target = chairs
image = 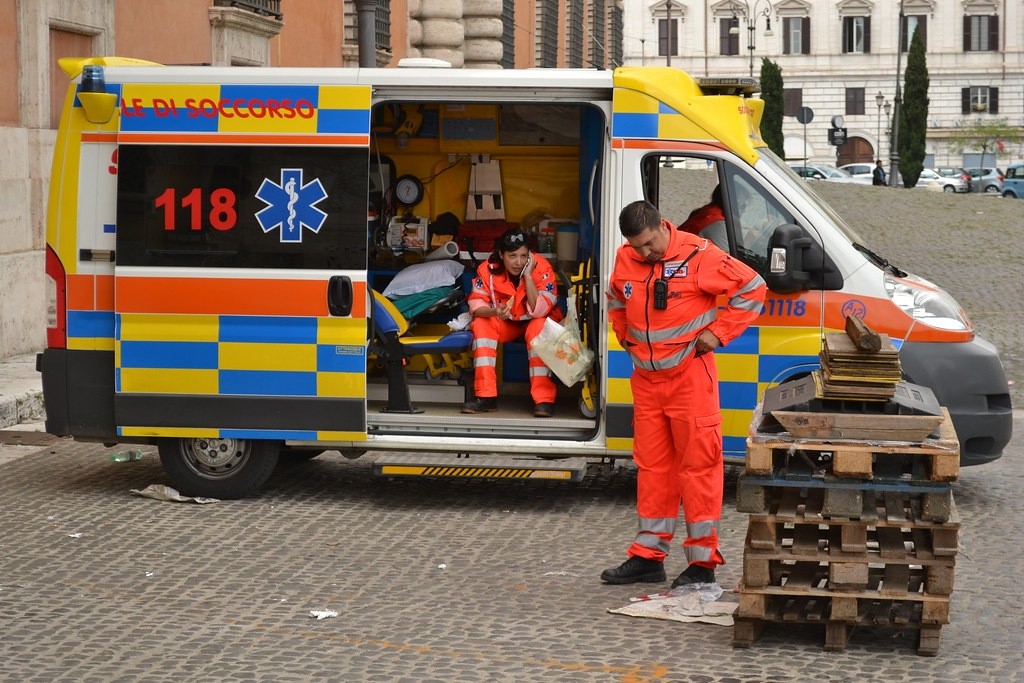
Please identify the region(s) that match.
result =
[369,285,472,383]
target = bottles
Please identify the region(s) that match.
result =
[544,236,553,253]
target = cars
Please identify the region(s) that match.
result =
[836,163,877,185]
[787,162,854,181]
[881,166,947,192]
[1001,162,1024,199]
[961,167,1005,194]
[933,167,970,193]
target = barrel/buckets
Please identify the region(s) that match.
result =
[555,226,579,261]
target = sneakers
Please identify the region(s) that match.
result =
[601,556,667,585]
[671,566,716,589]
[461,396,497,414]
[534,402,554,417]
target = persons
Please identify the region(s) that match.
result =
[873,160,886,186]
[463,229,563,417]
[677,180,750,253]
[604,201,767,590]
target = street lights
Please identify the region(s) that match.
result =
[640,33,649,65]
[875,91,884,161]
[883,100,892,161]
[729,1,775,78]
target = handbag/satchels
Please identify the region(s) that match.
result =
[531,305,596,388]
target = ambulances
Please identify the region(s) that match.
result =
[35,56,1014,501]
